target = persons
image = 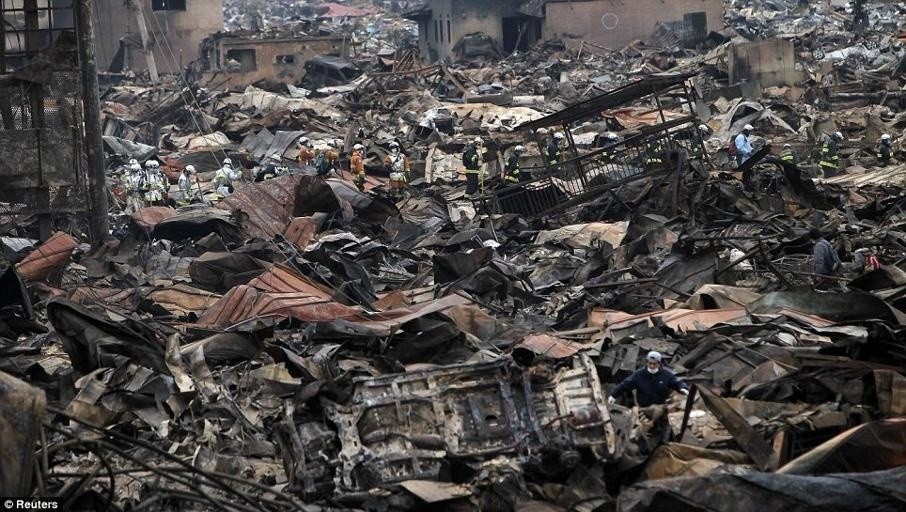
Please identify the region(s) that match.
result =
[120,158,204,214]
[294,133,345,177]
[821,129,845,180]
[212,157,244,196]
[810,133,828,162]
[733,124,756,167]
[608,350,690,444]
[779,142,796,163]
[383,141,411,194]
[259,153,287,183]
[646,138,663,164]
[351,142,369,192]
[874,135,896,165]
[464,135,485,199]
[501,144,526,188]
[690,122,714,161]
[804,225,843,284]
[545,129,566,178]
[595,143,628,166]
[796,155,825,179]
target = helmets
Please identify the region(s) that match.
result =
[129,132,618,174]
[698,124,792,149]
